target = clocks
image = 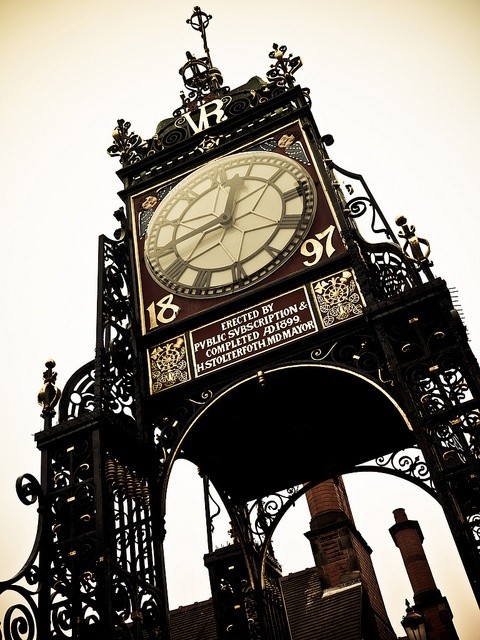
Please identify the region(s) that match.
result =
[129,117,350,336]
